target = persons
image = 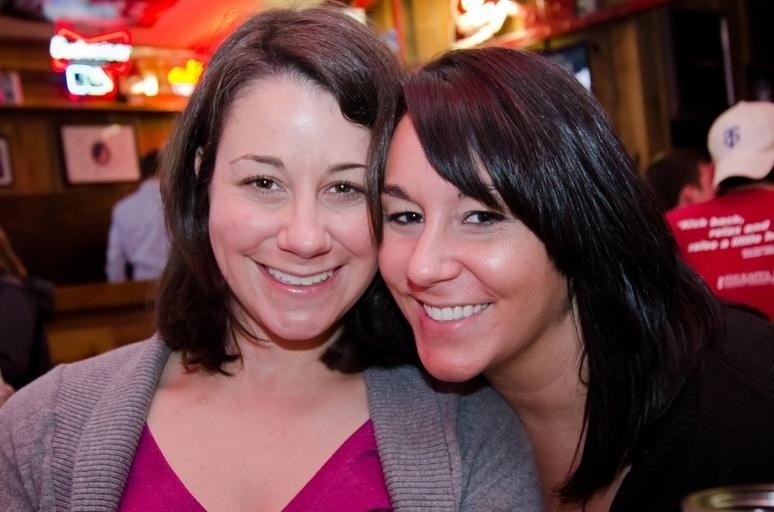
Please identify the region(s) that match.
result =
[105,148,176,282]
[364,44,774,512]
[646,148,716,219]
[665,96,774,326]
[2,6,548,512]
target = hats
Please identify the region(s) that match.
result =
[706,100,773,192]
